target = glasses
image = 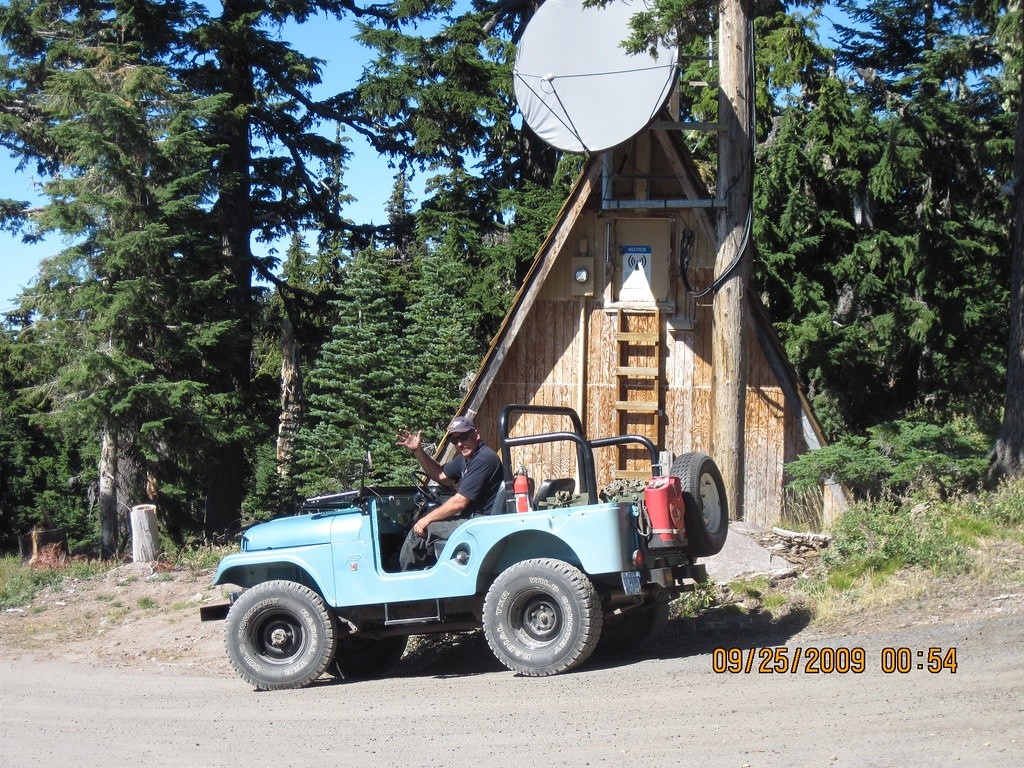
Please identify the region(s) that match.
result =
[450,430,474,445]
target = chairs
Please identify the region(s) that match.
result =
[531,478,576,512]
[432,477,535,561]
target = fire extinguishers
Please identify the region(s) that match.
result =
[513,464,533,513]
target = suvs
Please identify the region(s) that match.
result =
[197,404,731,692]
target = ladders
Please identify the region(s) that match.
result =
[613,307,661,482]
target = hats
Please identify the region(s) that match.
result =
[449,417,475,435]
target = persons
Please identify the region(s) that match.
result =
[393,417,504,572]
